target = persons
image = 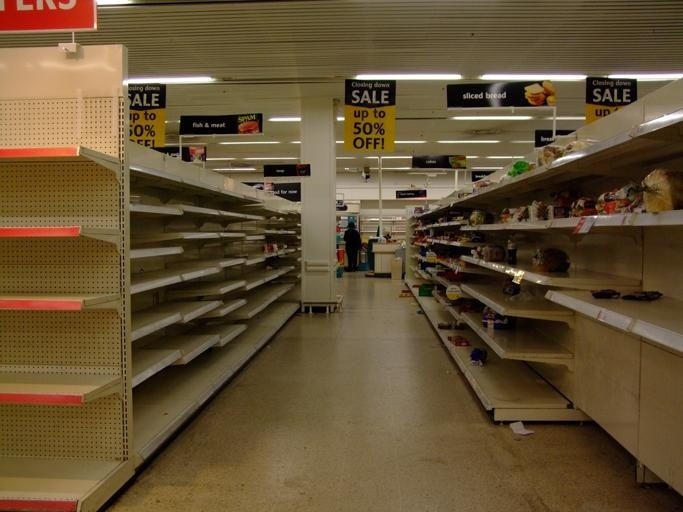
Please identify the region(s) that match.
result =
[344,222,362,272]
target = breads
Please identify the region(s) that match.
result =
[638,167,682,213]
[522,81,555,106]
[532,247,571,273]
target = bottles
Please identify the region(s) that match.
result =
[507,240,517,263]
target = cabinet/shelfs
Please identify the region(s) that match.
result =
[0,45,304,512]
[405,77,683,497]
[336,211,407,274]
